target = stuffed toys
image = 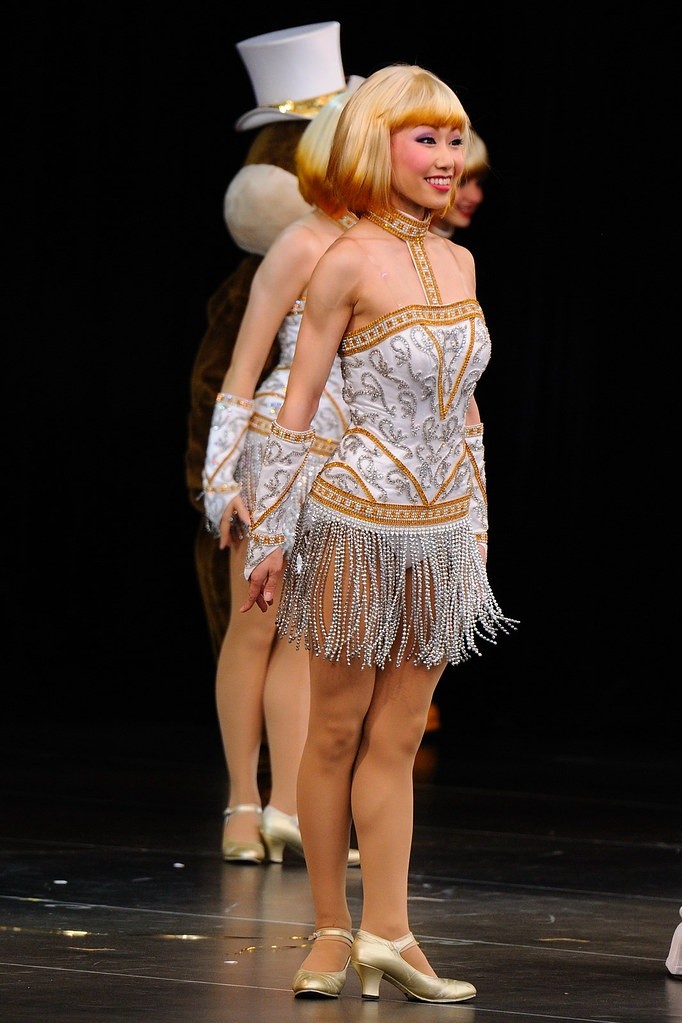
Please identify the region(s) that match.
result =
[184,20,346,657]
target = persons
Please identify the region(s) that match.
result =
[426,125,489,240]
[233,63,519,1004]
[197,87,366,870]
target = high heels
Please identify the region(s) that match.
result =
[291,925,355,998]
[352,929,477,1003]
[259,802,360,866]
[222,802,265,864]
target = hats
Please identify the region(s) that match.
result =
[236,20,351,132]
